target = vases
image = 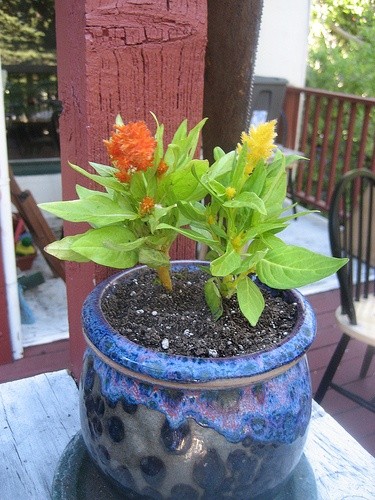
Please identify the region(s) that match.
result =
[79,260,318,500]
[17,247,37,271]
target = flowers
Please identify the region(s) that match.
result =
[37,109,348,328]
[16,237,35,256]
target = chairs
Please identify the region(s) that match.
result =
[4,81,62,157]
[272,110,298,221]
[315,168,375,412]
